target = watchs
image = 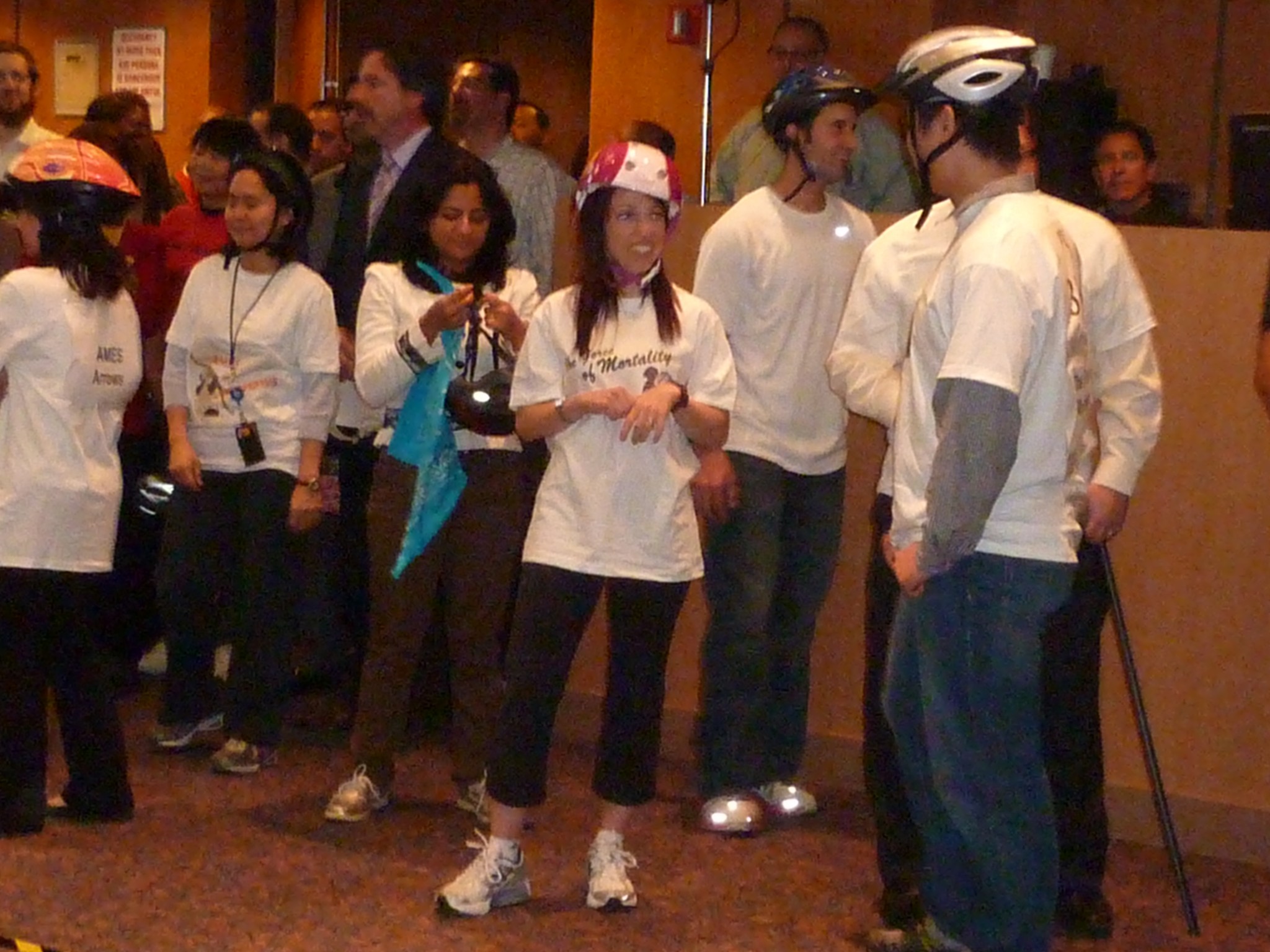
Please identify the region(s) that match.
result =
[300,478,322,491]
[556,399,576,424]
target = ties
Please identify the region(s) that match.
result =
[365,157,395,245]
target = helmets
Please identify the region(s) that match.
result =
[5,140,142,201]
[761,68,880,138]
[891,26,1037,109]
[574,139,683,219]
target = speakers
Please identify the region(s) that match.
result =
[1226,112,1270,232]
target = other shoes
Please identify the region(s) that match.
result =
[47,794,134,822]
[868,916,971,952]
[701,794,764,833]
[1057,888,1112,937]
[760,779,818,817]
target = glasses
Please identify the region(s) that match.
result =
[766,45,823,64]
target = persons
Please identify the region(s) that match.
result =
[1092,122,1202,226]
[705,17,915,210]
[324,149,543,828]
[828,103,1161,937]
[157,149,340,774]
[0,140,145,836]
[882,27,1082,952]
[0,35,580,726]
[691,65,880,826]
[432,139,738,913]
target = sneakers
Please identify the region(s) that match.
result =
[159,707,222,749]
[211,737,277,774]
[435,828,533,920]
[457,773,495,824]
[325,766,388,824]
[585,827,639,910]
